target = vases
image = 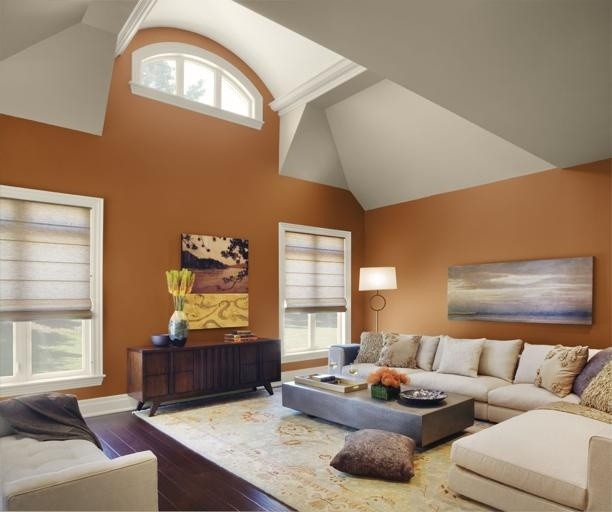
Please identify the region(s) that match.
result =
[168,311,189,347]
[371,384,401,400]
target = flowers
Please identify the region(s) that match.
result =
[364,368,411,389]
[166,268,195,311]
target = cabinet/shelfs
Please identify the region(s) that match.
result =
[128,338,281,416]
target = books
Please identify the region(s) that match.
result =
[224,330,258,344]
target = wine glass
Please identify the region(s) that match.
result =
[348,361,358,379]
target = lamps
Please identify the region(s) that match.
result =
[358,267,397,332]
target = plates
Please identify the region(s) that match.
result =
[399,389,449,404]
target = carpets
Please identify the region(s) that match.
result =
[131,388,506,512]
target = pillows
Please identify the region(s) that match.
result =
[329,427,417,483]
[581,362,612,415]
[437,336,485,378]
[514,342,602,384]
[535,344,589,397]
[571,347,612,398]
[478,339,522,382]
[375,332,422,368]
[354,332,383,363]
[414,336,440,372]
[432,335,445,371]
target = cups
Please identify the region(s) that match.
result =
[329,363,338,377]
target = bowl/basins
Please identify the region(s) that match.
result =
[151,334,170,346]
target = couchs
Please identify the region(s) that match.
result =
[446,406,612,512]
[329,332,612,421]
[0,394,159,512]
